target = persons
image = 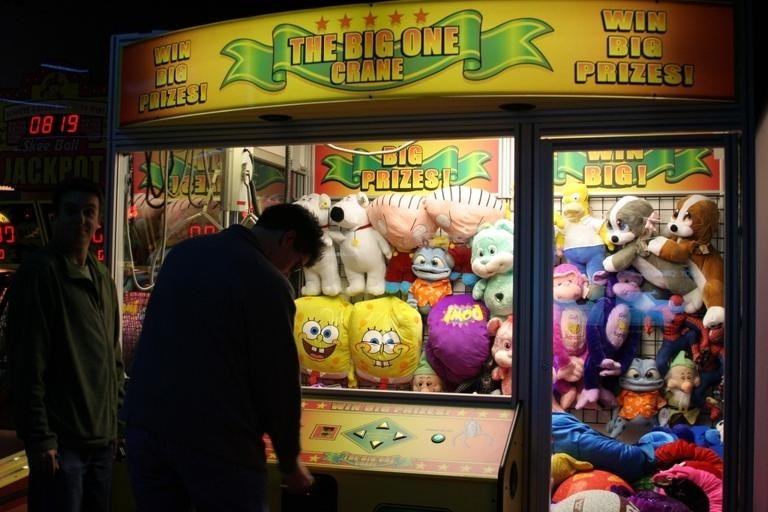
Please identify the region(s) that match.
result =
[2,177,130,510]
[120,200,327,510]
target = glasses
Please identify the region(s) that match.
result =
[290,243,303,273]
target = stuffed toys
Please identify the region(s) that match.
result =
[290,180,513,396]
[290,180,512,396]
[551,183,722,512]
[551,174,723,512]
